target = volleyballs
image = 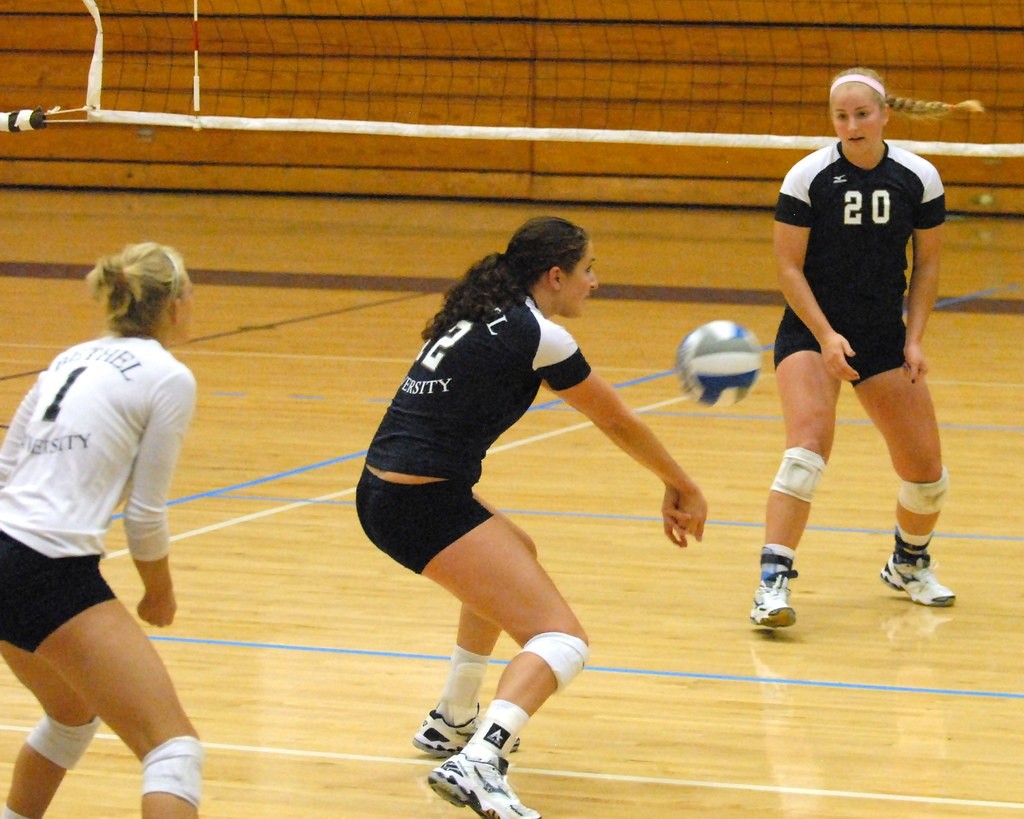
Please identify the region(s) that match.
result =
[674,319,765,409]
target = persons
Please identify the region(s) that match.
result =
[357,215,706,817]
[1,241,198,819]
[746,66,982,631]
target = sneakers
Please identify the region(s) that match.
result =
[750,569,799,627]
[413,708,521,756]
[880,551,956,607]
[427,752,540,819]
